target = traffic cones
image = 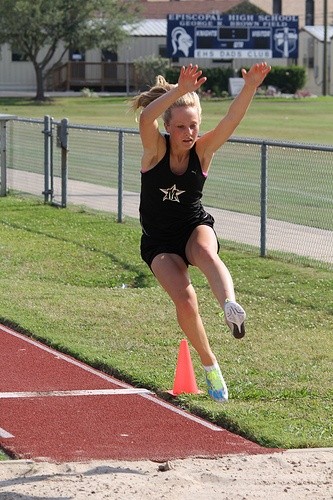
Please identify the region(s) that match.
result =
[166,339,209,401]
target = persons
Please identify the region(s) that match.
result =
[131,61,273,402]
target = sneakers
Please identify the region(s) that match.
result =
[222,301,246,338]
[200,355,228,403]
